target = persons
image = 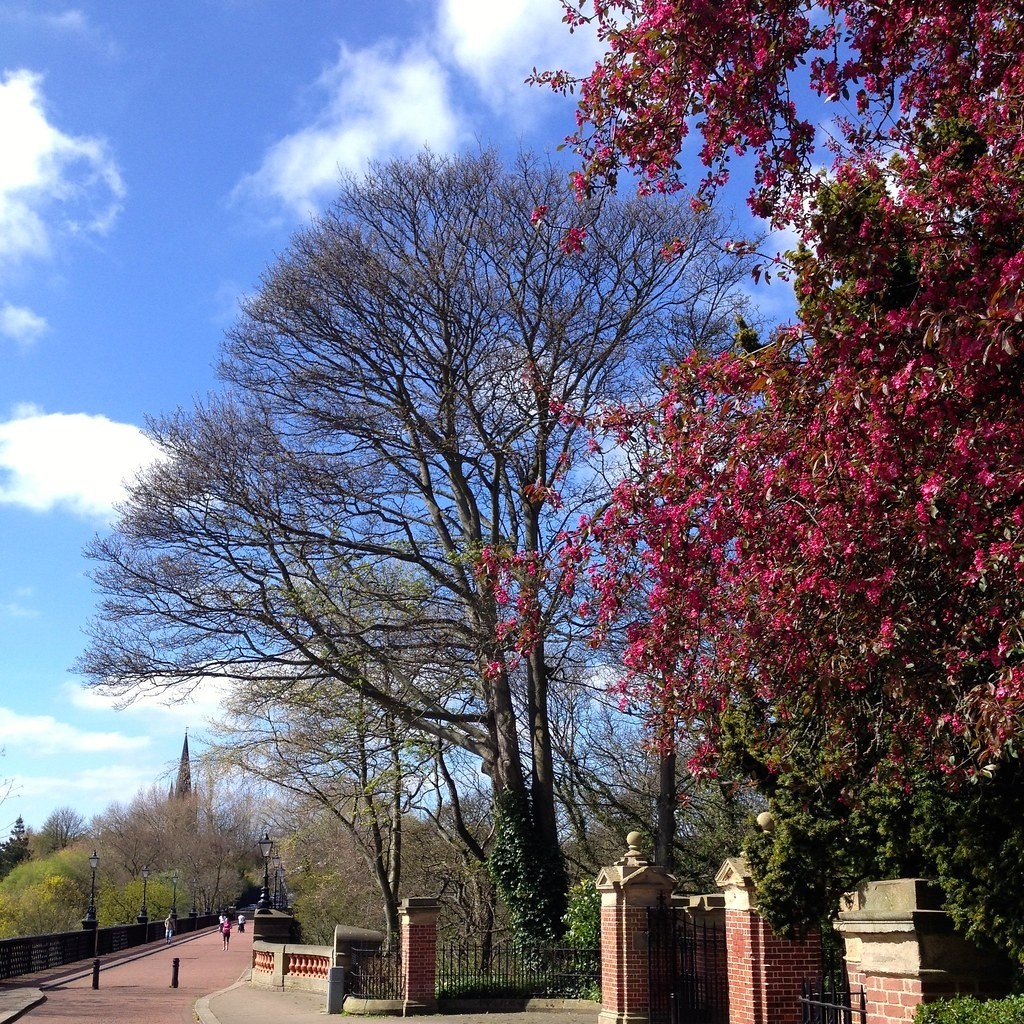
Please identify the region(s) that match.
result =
[238,913,245,933]
[219,912,226,934]
[165,913,175,944]
[222,918,232,951]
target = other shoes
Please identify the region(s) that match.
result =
[166,941,171,944]
[222,948,228,951]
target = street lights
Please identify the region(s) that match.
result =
[169,872,179,916]
[85,849,101,921]
[257,832,274,908]
[139,863,149,916]
[271,849,288,910]
[190,876,199,912]
